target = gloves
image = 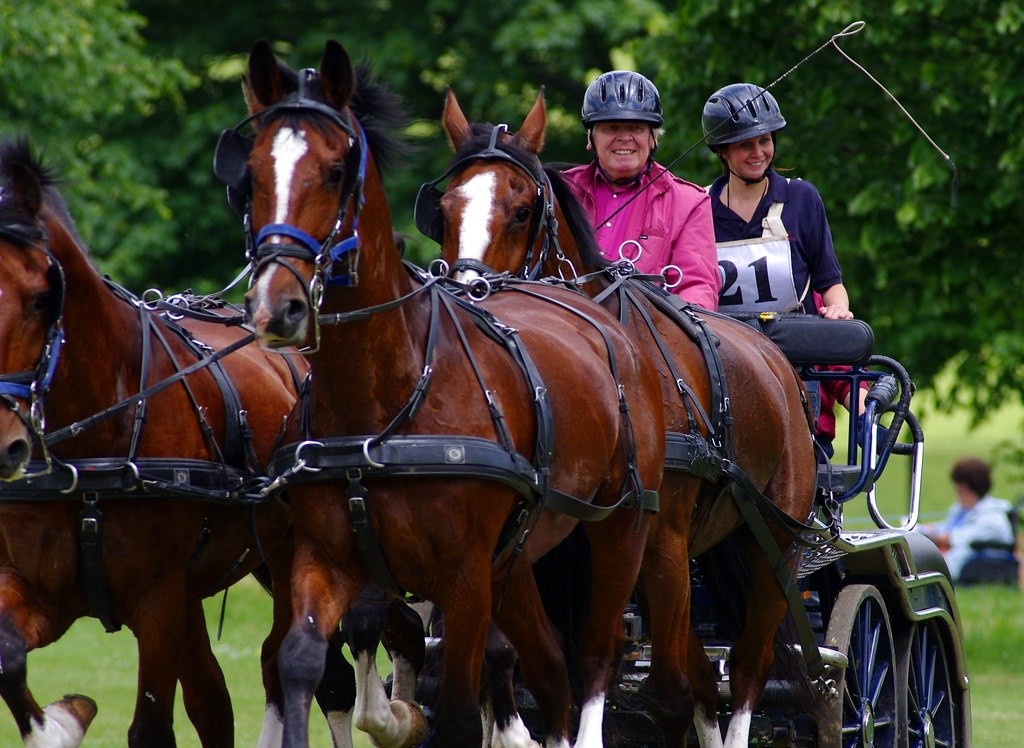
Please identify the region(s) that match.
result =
[857,413,888,455]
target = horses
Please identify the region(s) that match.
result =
[438,80,815,748]
[215,37,661,745]
[1,128,429,748]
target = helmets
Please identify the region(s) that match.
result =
[701,84,786,153]
[581,71,664,130]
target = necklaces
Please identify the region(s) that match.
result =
[723,176,768,208]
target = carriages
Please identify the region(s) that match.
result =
[0,39,974,747]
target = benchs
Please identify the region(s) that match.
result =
[762,318,875,493]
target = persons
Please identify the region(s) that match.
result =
[915,459,1024,586]
[697,81,855,423]
[554,70,720,315]
[814,290,868,464]
